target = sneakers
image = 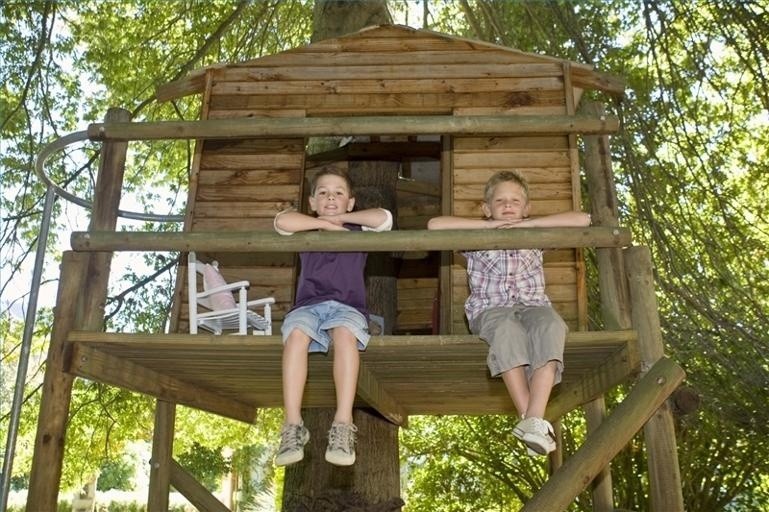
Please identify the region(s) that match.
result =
[325,420,358,466]
[275,420,310,467]
[512,417,556,456]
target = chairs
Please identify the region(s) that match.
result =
[187,252,276,336]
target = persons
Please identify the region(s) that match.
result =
[426,169,592,457]
[272,163,394,467]
